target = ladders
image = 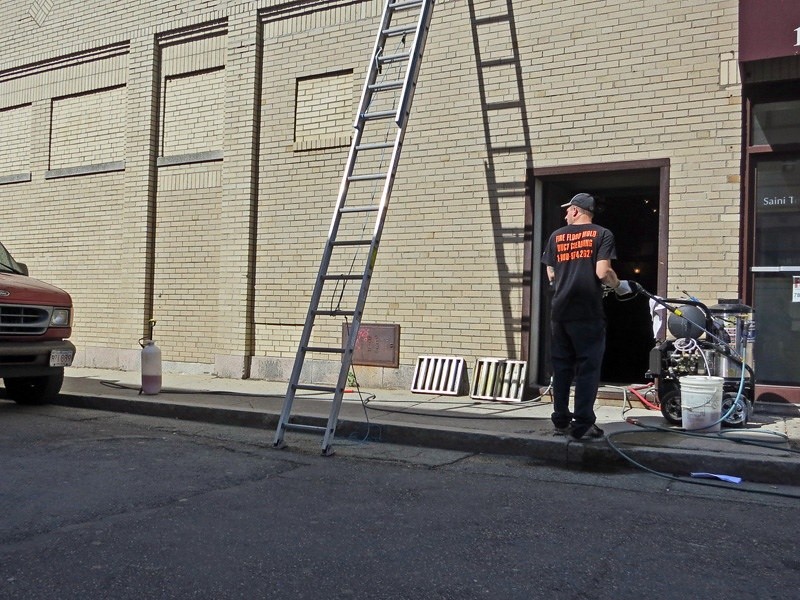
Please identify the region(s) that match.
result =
[274,0,434,456]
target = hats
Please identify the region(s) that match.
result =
[561,193,595,213]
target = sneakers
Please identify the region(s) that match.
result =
[581,423,604,439]
[554,423,572,435]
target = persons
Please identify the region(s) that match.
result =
[541,193,633,437]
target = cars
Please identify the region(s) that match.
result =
[0,241,75,405]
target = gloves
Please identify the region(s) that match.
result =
[614,280,633,296]
